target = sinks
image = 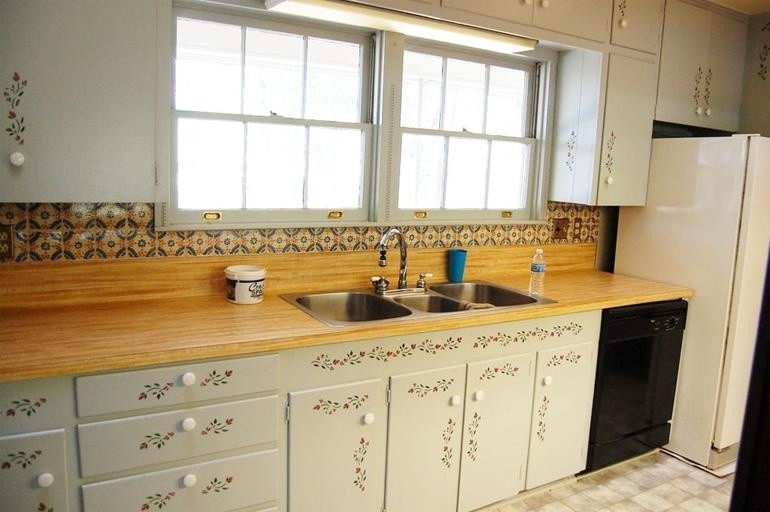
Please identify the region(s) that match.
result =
[391,290,476,314]
[288,288,417,326]
[427,280,539,307]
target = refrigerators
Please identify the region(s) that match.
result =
[614,133,769,474]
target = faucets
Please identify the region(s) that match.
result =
[377,227,409,288]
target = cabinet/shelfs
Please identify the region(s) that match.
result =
[0,0,172,203]
[353,0,664,60]
[524,311,603,491]
[749,11,769,136]
[547,48,660,209]
[655,3,747,132]
[75,350,289,512]
[0,376,78,512]
[288,337,389,511]
[388,318,538,512]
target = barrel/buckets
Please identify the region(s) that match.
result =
[223,264,267,304]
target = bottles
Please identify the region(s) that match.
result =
[529,248,545,296]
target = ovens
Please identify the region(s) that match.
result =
[582,295,691,481]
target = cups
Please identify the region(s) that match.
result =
[447,249,467,283]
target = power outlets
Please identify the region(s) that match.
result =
[574,217,581,239]
[553,218,568,240]
